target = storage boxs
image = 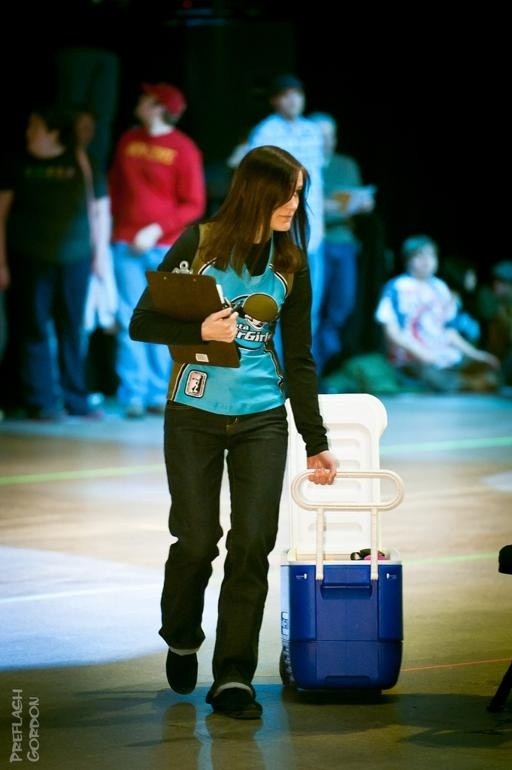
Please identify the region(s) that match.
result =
[278,391,406,703]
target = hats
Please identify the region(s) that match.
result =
[141,83,187,118]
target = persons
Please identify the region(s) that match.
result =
[125,144,340,721]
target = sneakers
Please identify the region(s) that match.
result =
[211,685,263,719]
[164,646,198,695]
